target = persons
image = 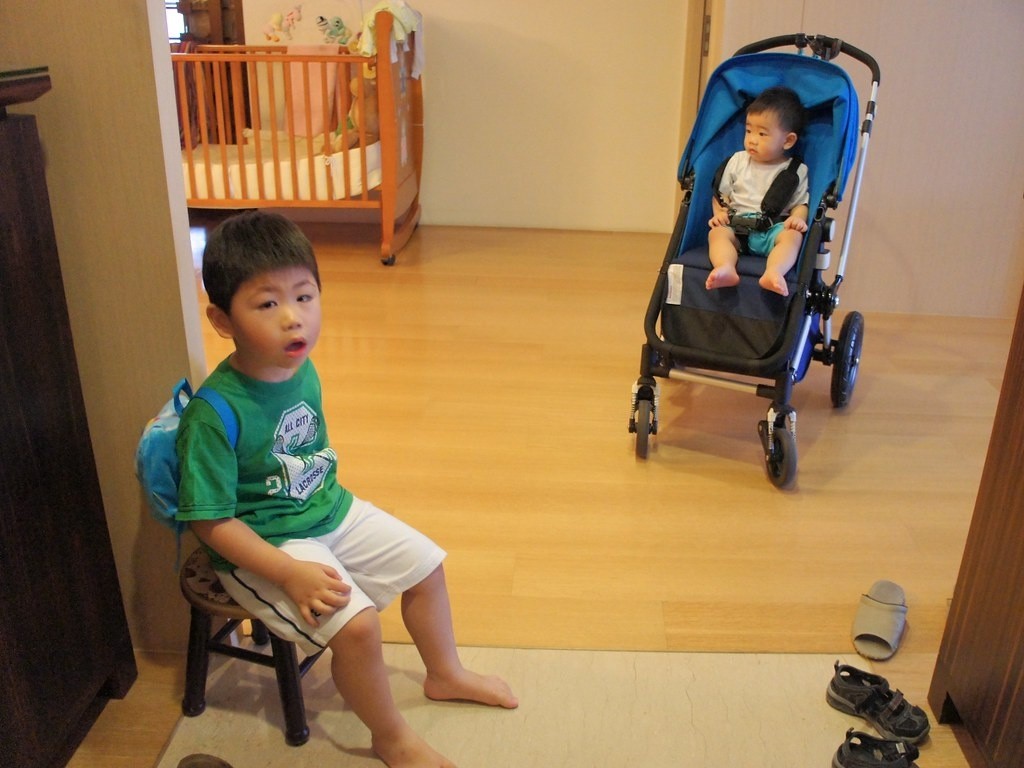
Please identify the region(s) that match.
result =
[704,84,812,294]
[170,208,521,768]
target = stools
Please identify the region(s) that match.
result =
[179,545,310,747]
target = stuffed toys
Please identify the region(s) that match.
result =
[323,61,381,155]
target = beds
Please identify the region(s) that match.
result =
[165,0,425,265]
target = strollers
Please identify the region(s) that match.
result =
[626,31,883,490]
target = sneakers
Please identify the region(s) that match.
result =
[827,662,931,768]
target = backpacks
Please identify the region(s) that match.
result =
[137,377,240,531]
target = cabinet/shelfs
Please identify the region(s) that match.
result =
[0,65,142,767]
[927,287,1024,768]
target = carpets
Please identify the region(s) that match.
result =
[150,640,885,767]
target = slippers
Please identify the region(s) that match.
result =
[851,581,908,661]
